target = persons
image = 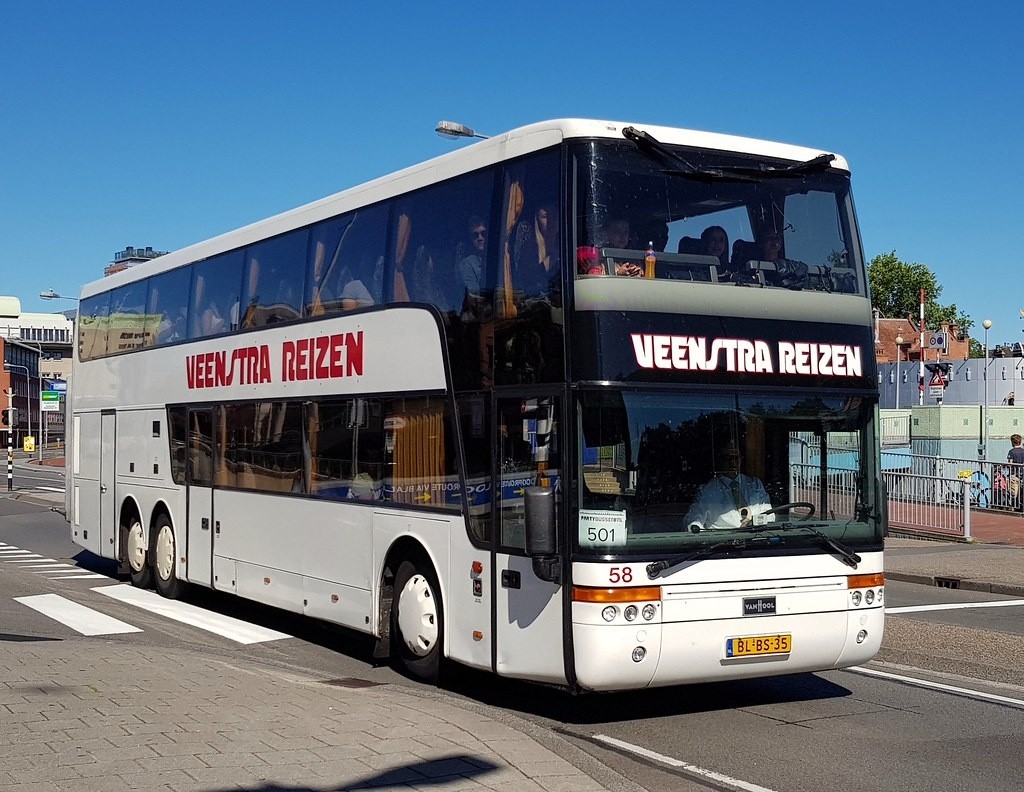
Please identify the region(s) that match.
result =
[1007,434,1024,512]
[682,435,776,531]
[351,472,384,500]
[156,201,784,344]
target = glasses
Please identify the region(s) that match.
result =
[471,231,487,239]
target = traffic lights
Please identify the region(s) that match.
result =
[926,363,952,373]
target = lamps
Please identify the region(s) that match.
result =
[903,359,918,382]
[985,348,1004,371]
[957,344,982,374]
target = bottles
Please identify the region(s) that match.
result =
[645,241,656,279]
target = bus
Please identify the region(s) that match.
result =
[65,116,885,693]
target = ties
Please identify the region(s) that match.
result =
[731,481,752,520]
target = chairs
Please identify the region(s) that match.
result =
[168,235,754,337]
[192,432,382,499]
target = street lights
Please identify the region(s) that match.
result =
[4,363,31,436]
[895,337,903,409]
[982,319,992,461]
[8,336,42,467]
